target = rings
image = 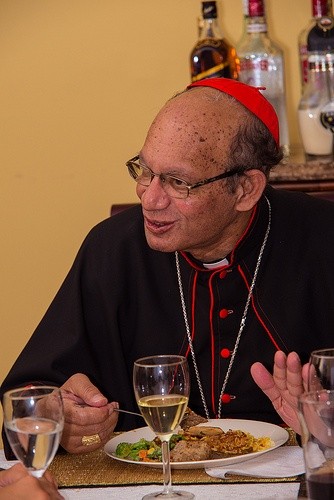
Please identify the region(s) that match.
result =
[82,434,102,446]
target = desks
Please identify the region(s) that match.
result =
[0,423,334,500]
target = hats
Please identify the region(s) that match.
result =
[187,77,279,147]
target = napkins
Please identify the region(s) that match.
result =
[206,441,326,480]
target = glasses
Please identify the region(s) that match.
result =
[126,155,238,199]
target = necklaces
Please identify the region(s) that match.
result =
[175,196,271,419]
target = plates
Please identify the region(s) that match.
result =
[104,419,290,469]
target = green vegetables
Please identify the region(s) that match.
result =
[115,434,183,460]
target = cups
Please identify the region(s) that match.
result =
[307,348,334,430]
[3,386,64,479]
[296,390,334,500]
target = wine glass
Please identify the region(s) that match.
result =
[133,355,195,500]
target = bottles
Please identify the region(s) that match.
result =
[189,0,240,83]
[298,0,334,156]
[237,0,291,165]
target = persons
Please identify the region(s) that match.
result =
[0,79,334,463]
